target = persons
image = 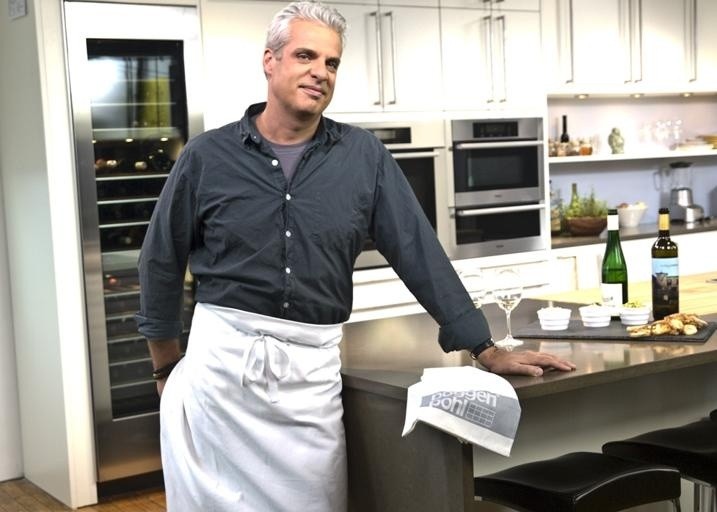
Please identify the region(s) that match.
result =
[133,1,577,512]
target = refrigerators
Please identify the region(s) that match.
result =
[60,4,211,489]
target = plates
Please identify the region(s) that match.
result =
[694,134,716,150]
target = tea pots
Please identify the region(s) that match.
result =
[667,119,685,152]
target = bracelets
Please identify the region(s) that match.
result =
[469,337,493,361]
[153,354,184,380]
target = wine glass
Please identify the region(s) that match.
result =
[455,269,488,312]
[488,269,526,348]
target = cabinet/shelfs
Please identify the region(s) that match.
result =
[601,1,661,95]
[313,1,445,117]
[439,0,547,117]
[346,265,434,325]
[661,1,716,95]
[543,0,600,95]
[457,258,550,298]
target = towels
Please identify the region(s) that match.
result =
[399,365,522,458]
[574,254,602,290]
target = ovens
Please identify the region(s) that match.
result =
[447,118,547,255]
[351,122,451,270]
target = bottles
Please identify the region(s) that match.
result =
[600,207,626,314]
[652,207,678,318]
[569,137,580,156]
[579,136,592,155]
[548,137,555,157]
[94,141,182,174]
[553,138,559,157]
[560,116,570,156]
[549,179,564,234]
[100,270,121,289]
[567,183,579,209]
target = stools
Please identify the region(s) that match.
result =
[604,413,716,511]
[473,445,681,510]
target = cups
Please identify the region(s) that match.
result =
[578,305,610,328]
[616,303,652,326]
[536,307,572,331]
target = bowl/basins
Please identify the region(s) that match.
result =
[616,204,647,228]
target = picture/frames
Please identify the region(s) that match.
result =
[6,0,27,21]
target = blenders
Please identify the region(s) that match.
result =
[666,162,702,222]
